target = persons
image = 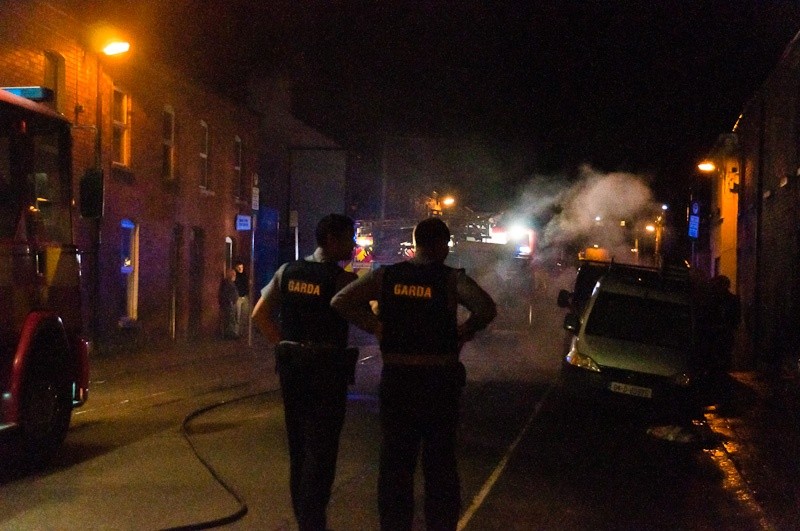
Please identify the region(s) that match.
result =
[251,214,375,530]
[710,275,740,417]
[330,218,497,531]
[218,260,250,340]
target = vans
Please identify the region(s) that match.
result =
[558,255,719,424]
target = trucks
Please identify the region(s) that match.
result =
[0,86,103,479]
